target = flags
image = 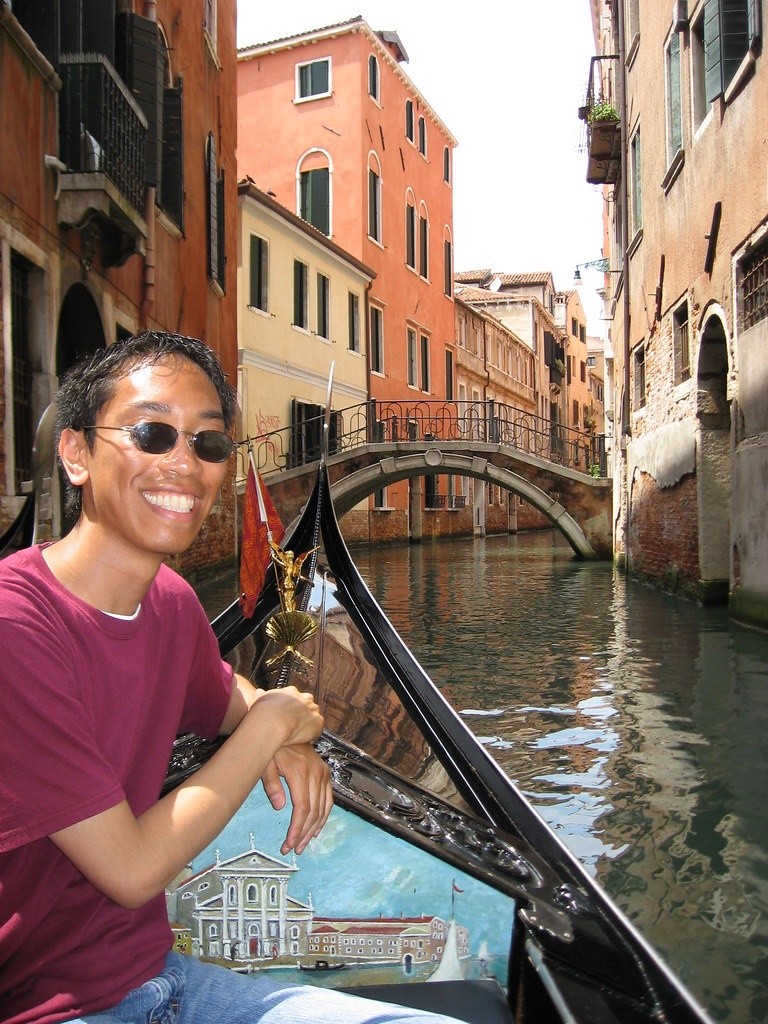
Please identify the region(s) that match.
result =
[238,460,286,619]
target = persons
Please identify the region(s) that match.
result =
[270,551,295,612]
[0,330,470,1024]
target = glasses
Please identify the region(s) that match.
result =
[79,421,240,464]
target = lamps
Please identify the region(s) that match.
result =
[572,258,609,286]
